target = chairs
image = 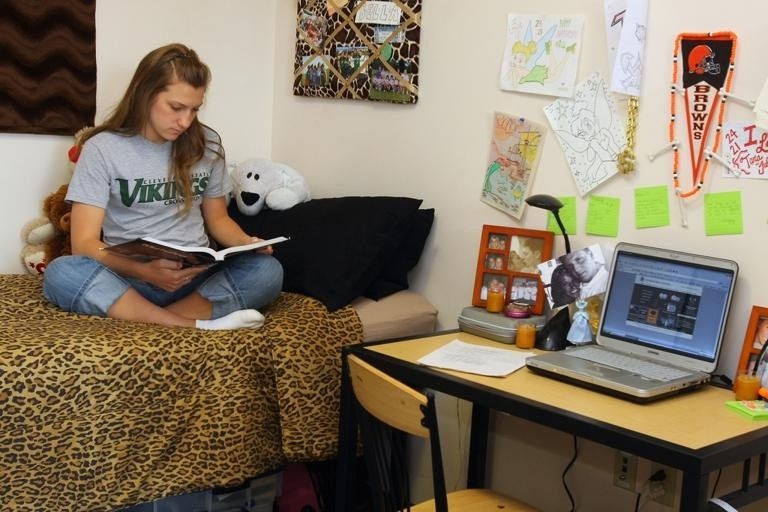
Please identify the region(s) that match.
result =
[341,348,544,512]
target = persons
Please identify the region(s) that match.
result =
[560,248,610,299]
[41,42,283,331]
[480,231,543,302]
[746,354,759,375]
[752,315,768,350]
[551,263,581,309]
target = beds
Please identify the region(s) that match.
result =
[2,272,438,512]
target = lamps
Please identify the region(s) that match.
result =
[525,195,573,353]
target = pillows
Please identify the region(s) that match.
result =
[227,188,435,315]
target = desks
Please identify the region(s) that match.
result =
[358,329,768,512]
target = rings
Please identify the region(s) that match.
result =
[185,275,190,281]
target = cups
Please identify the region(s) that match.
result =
[733,372,761,403]
[485,280,507,314]
[515,322,538,350]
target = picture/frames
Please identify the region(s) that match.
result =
[471,224,554,317]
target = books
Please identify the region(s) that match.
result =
[98,236,291,265]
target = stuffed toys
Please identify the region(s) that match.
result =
[18,126,94,282]
[225,157,313,219]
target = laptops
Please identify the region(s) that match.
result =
[525,242,739,404]
